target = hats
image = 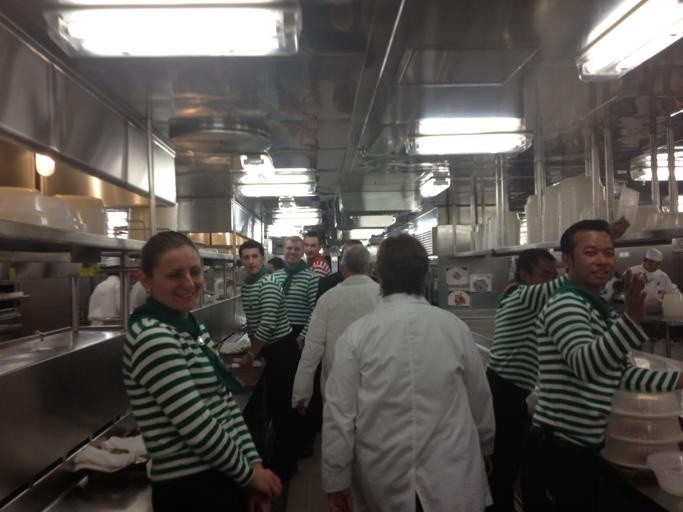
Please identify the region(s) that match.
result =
[646,248,663,261]
[104,257,120,266]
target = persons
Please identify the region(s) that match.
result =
[319,234,496,512]
[522,221,649,512]
[622,247,681,305]
[129,280,148,314]
[240,238,301,441]
[486,216,631,512]
[122,231,282,512]
[262,230,378,358]
[87,257,121,325]
[214,279,236,300]
[290,243,382,416]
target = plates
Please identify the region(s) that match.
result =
[75,453,152,483]
